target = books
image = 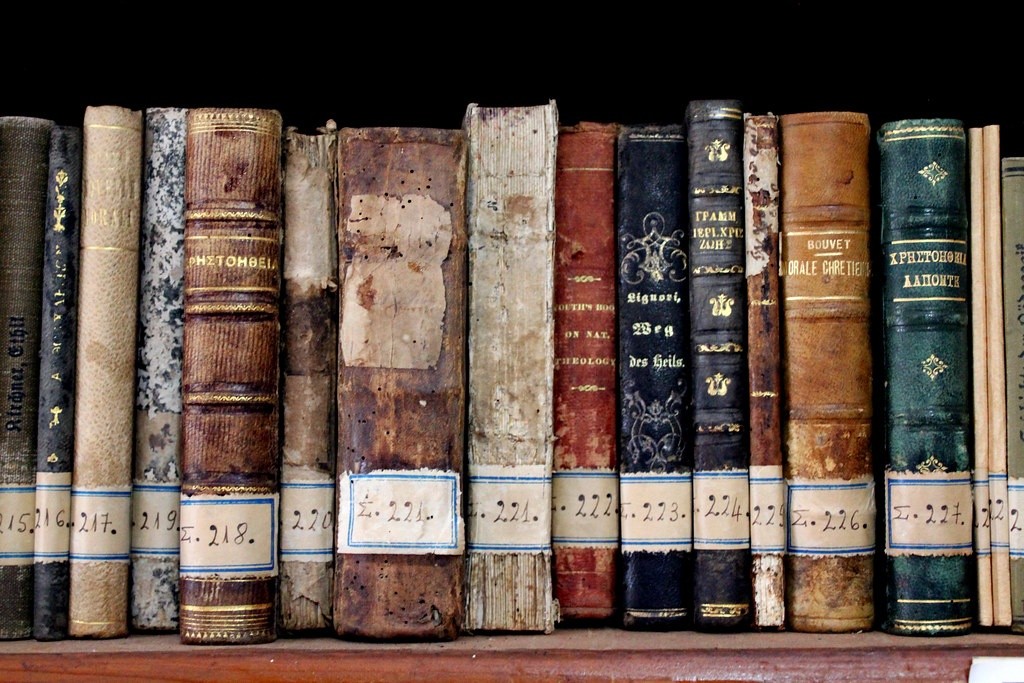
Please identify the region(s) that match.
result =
[1,103,1024,646]
